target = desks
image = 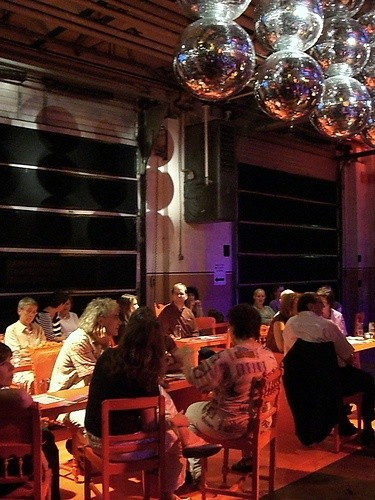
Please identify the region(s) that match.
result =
[175,333,227,374]
[346,337,375,352]
[31,374,195,416]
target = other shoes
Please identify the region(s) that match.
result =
[183,438,222,457]
[341,424,359,436]
[360,429,375,442]
[232,458,252,473]
[173,477,208,498]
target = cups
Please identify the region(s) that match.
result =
[369,322,375,339]
[175,325,181,340]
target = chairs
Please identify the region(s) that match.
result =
[0,303,363,500]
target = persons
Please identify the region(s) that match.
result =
[34,291,69,343]
[57,293,79,336]
[266,289,297,354]
[316,288,347,336]
[186,286,203,318]
[85,305,224,500]
[113,293,139,343]
[47,297,122,391]
[158,282,199,338]
[253,288,276,322]
[270,285,288,314]
[186,305,278,497]
[0,343,60,500]
[3,297,68,429]
[284,293,375,447]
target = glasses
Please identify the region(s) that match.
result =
[22,309,38,316]
[316,301,322,305]
